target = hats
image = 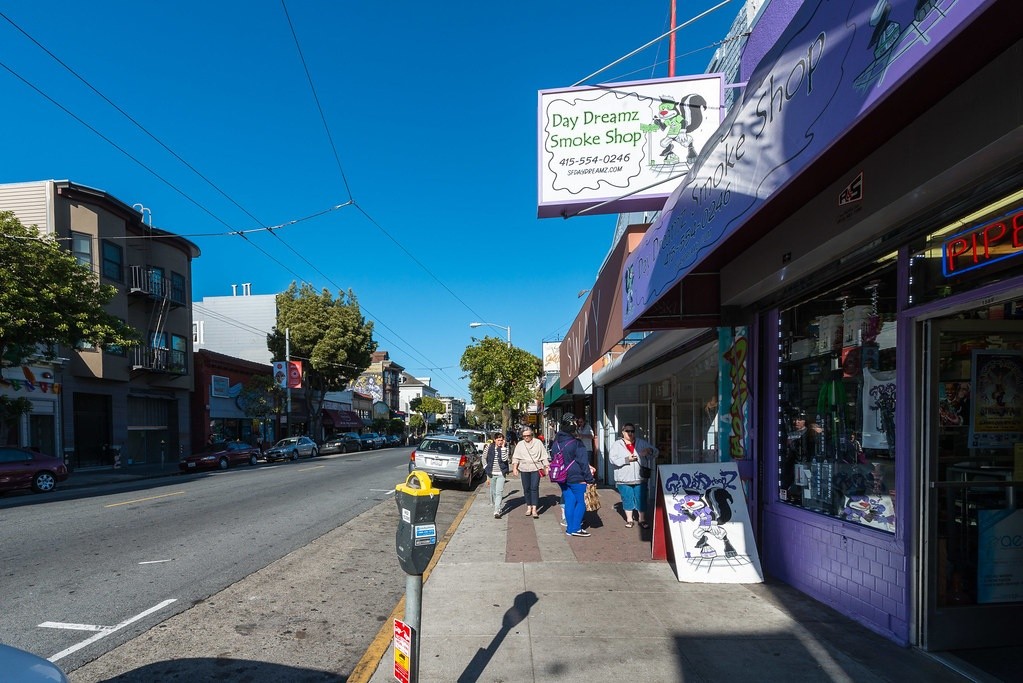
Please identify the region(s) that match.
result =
[561,418,576,432]
[563,413,578,421]
[523,427,533,436]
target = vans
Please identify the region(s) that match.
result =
[453,428,487,453]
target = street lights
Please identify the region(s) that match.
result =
[470,322,511,348]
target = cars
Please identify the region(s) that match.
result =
[382,435,401,448]
[178,440,263,470]
[325,432,362,453]
[408,435,485,489]
[263,436,319,461]
[0,445,69,493]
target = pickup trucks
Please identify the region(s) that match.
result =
[360,433,386,450]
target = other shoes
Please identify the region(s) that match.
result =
[494,511,500,518]
[532,511,538,518]
[525,510,532,515]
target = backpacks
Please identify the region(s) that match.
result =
[548,438,577,483]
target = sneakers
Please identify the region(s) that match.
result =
[566,529,591,536]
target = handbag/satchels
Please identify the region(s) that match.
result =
[639,466,652,478]
[538,469,546,477]
[584,483,602,512]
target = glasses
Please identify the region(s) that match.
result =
[625,430,635,433]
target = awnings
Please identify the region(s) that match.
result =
[354,392,374,401]
[321,409,363,427]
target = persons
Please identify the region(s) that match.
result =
[940,381,971,417]
[257,435,263,451]
[786,410,820,500]
[378,430,382,437]
[512,427,549,517]
[208,434,216,448]
[292,428,314,441]
[551,413,595,536]
[482,432,509,518]
[850,432,863,456]
[703,396,718,450]
[224,436,233,442]
[609,423,659,530]
[359,427,362,436]
[808,422,826,461]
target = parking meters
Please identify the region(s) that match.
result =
[395,470,441,683]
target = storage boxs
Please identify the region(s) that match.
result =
[790,337,817,361]
[818,313,843,352]
[842,304,872,377]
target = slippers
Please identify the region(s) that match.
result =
[625,521,634,528]
[637,520,649,529]
